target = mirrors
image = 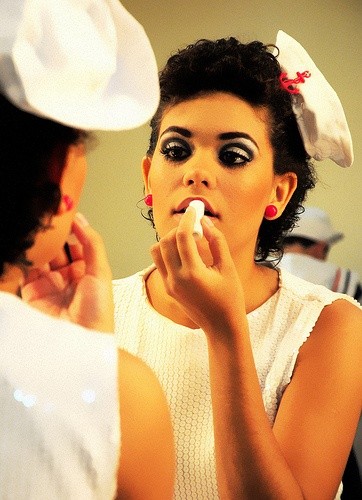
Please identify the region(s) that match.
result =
[75,0,362,500]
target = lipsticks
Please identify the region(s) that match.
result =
[187,200,206,239]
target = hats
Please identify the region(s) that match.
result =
[273,28,354,170]
[281,207,345,244]
[0,0,159,133]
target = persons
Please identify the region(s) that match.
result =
[110,30,362,500]
[1,0,177,500]
